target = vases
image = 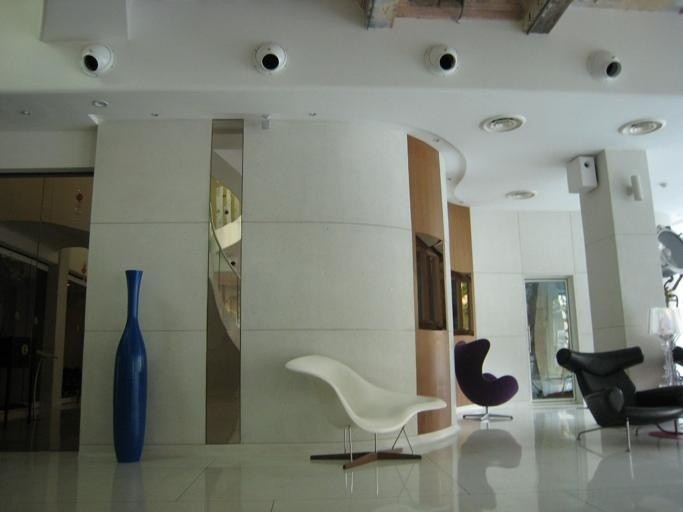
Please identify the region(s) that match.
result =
[110,267,149,464]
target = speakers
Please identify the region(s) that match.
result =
[567,155,598,193]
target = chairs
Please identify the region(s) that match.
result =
[555,338,682,460]
[453,333,518,426]
[283,344,450,473]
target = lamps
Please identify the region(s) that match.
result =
[624,173,642,203]
[646,303,683,440]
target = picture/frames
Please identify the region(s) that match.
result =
[450,269,475,336]
[413,232,447,332]
